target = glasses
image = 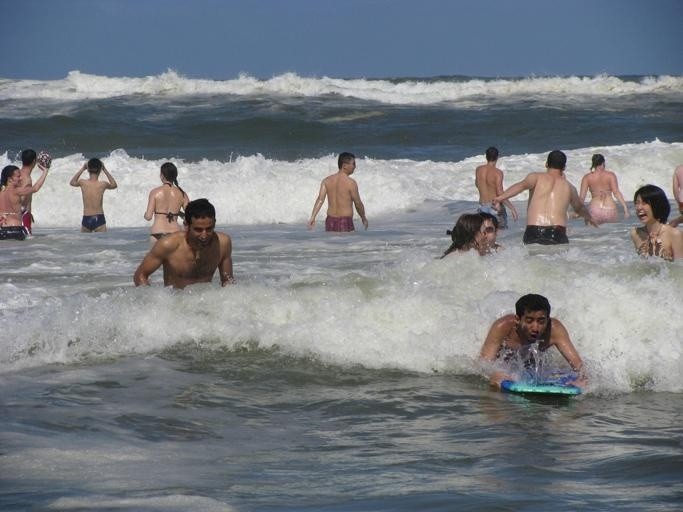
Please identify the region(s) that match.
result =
[486,226,495,234]
[344,162,356,165]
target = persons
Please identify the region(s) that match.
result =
[669,216,682,228]
[482,293,586,395]
[1,161,52,239]
[474,147,521,221]
[441,214,489,259]
[17,148,35,235]
[70,157,118,232]
[472,213,501,252]
[145,162,189,241]
[133,199,234,291]
[668,165,683,227]
[493,149,599,245]
[632,185,683,261]
[307,153,370,234]
[580,154,629,224]
